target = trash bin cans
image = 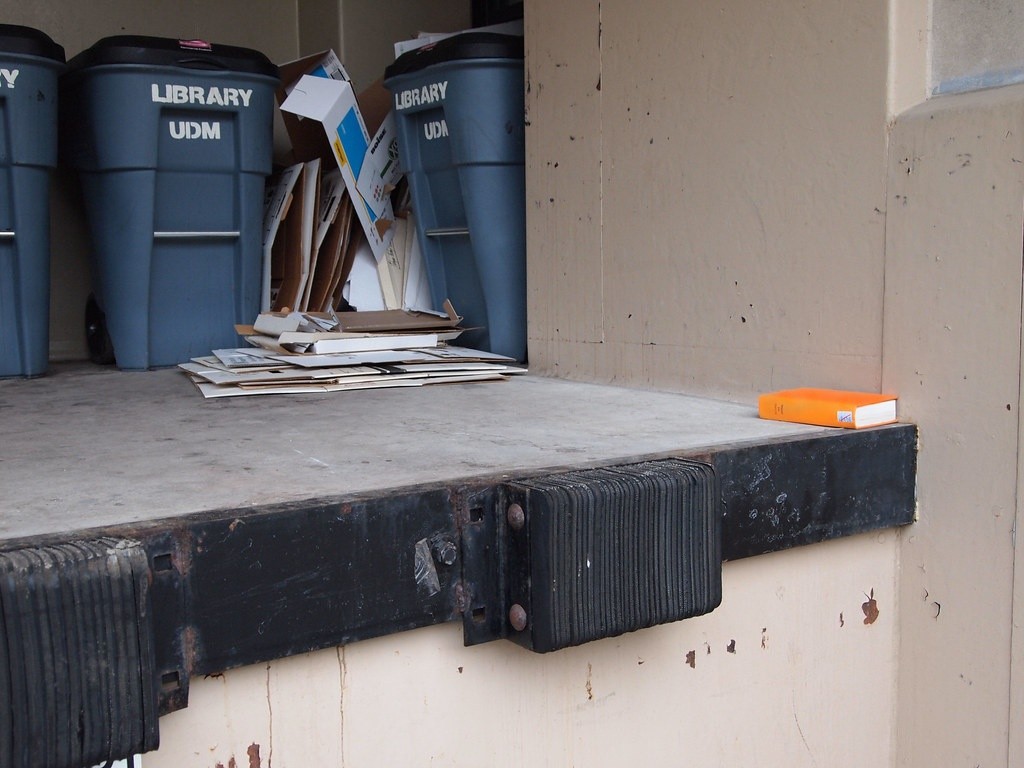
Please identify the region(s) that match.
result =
[64,32,282,374]
[0,23,68,383]
[380,31,526,364]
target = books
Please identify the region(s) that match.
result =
[758,385,900,430]
